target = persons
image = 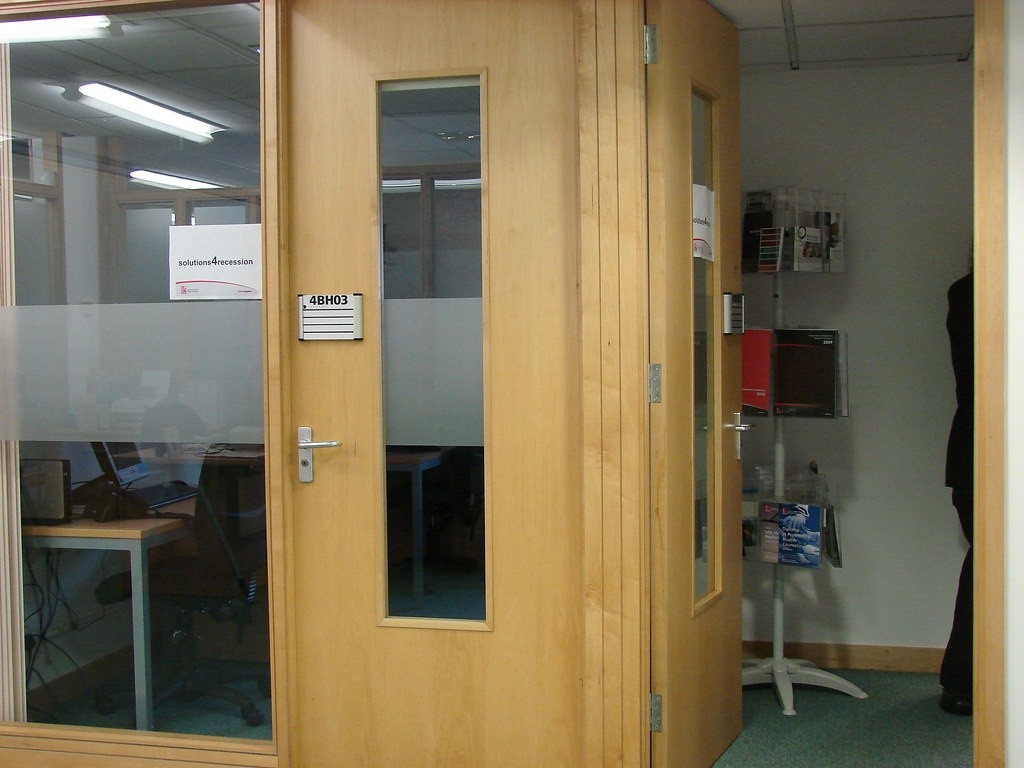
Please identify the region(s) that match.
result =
[940,271,974,717]
[802,242,821,258]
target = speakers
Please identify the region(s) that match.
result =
[18,458,70,525]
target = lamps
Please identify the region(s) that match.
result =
[63,81,230,147]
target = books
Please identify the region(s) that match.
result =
[742,326,850,419]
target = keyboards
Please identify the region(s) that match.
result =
[130,480,198,510]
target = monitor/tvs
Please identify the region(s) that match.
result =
[71,441,150,513]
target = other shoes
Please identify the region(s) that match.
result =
[939,689,973,714]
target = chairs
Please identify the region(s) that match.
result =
[94,443,272,727]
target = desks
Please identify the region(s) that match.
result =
[386,445,452,604]
[22,486,197,731]
